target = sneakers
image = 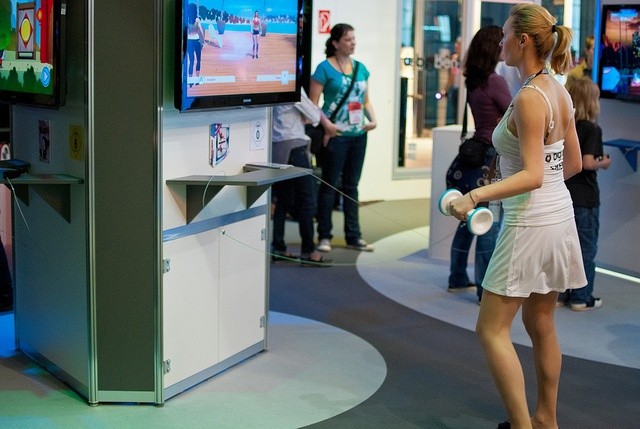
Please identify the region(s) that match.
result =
[448,283,477,293]
[272,251,300,263]
[300,256,334,267]
[345,239,374,251]
[556,300,567,307]
[317,238,331,251]
[572,297,602,311]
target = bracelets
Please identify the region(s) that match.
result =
[469,192,476,205]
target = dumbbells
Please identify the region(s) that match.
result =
[438,188,494,237]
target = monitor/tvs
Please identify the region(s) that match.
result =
[174,0,303,114]
[1,0,68,110]
[596,3,639,104]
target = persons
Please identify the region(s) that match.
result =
[448,26,514,301]
[555,77,611,312]
[564,35,595,91]
[272,79,344,266]
[188,4,205,86]
[441,37,462,123]
[475,3,589,429]
[309,23,376,252]
[251,11,262,58]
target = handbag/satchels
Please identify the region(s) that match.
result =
[305,123,326,155]
[445,138,497,194]
